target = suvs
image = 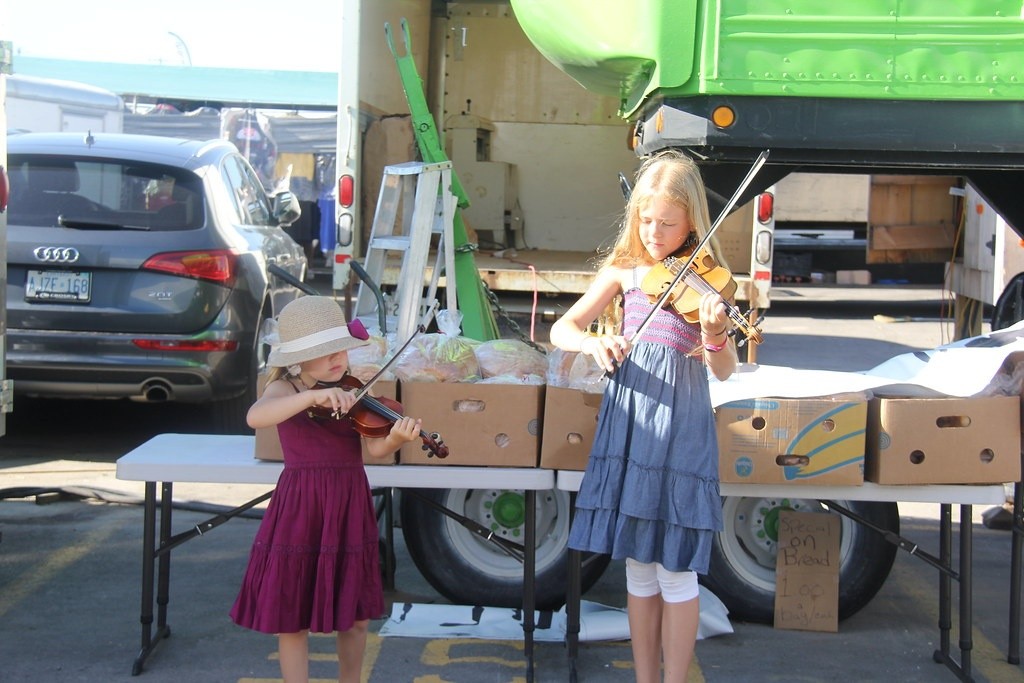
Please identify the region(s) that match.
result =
[4,130,308,436]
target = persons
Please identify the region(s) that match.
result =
[549,150,737,683]
[228,295,424,683]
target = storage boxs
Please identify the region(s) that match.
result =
[865,394,1021,484]
[836,270,872,286]
[254,370,400,466]
[713,394,868,487]
[400,382,545,469]
[540,382,604,471]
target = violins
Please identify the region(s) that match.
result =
[638,240,767,347]
[306,372,450,459]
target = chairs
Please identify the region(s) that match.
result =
[155,179,191,226]
[18,162,93,225]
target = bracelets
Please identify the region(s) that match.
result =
[700,327,726,337]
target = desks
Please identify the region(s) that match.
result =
[114,433,556,683]
[555,469,1007,683]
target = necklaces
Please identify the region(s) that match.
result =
[296,372,311,390]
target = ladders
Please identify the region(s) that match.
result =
[349,160,459,343]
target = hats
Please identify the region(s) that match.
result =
[267,295,370,368]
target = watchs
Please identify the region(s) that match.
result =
[702,334,729,352]
